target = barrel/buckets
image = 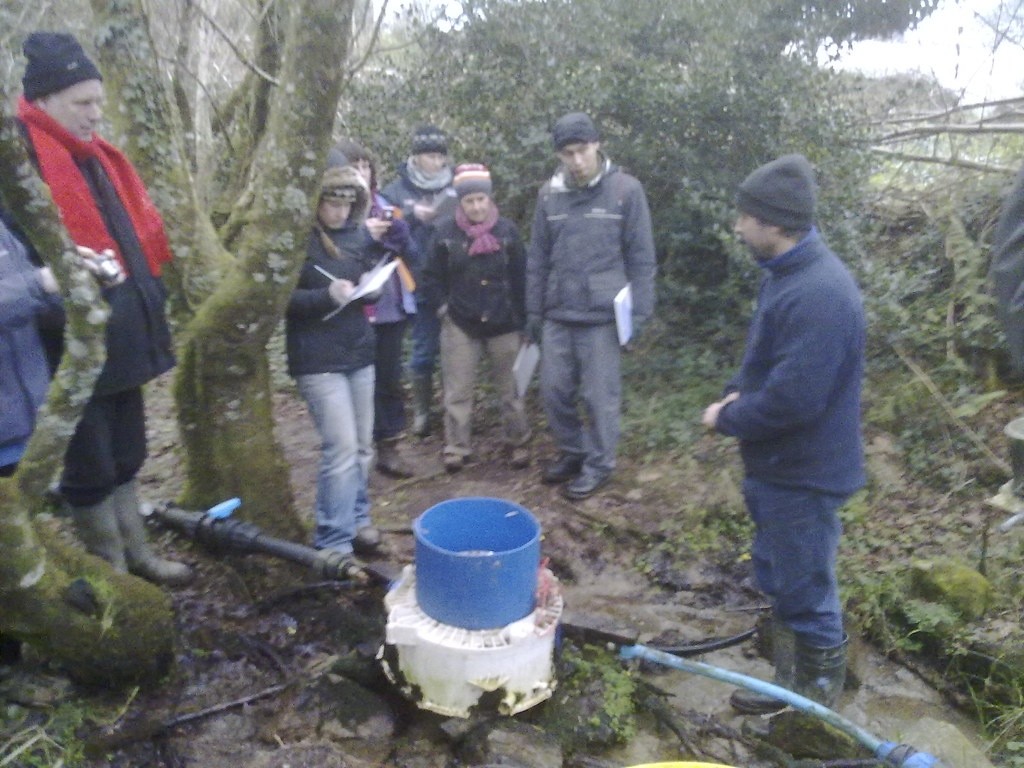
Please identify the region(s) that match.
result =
[409,496,543,632]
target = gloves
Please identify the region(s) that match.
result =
[388,219,410,242]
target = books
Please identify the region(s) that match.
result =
[613,283,634,347]
[322,251,401,322]
[512,341,541,398]
[432,187,457,210]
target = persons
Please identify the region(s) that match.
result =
[0,32,194,679]
[285,126,457,581]
[525,111,656,500]
[701,155,866,742]
[422,162,527,472]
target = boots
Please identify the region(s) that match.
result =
[411,372,433,433]
[729,625,852,740]
[70,480,195,591]
[376,435,414,478]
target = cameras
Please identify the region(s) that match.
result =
[380,206,394,222]
[89,255,118,283]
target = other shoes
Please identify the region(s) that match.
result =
[356,526,380,545]
[442,455,466,470]
[510,447,530,468]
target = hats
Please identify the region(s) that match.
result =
[23,32,103,103]
[411,127,449,155]
[553,112,600,147]
[453,163,492,200]
[733,155,817,231]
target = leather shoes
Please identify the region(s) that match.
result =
[541,454,585,481]
[564,465,613,499]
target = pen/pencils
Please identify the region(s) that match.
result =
[314,265,338,281]
